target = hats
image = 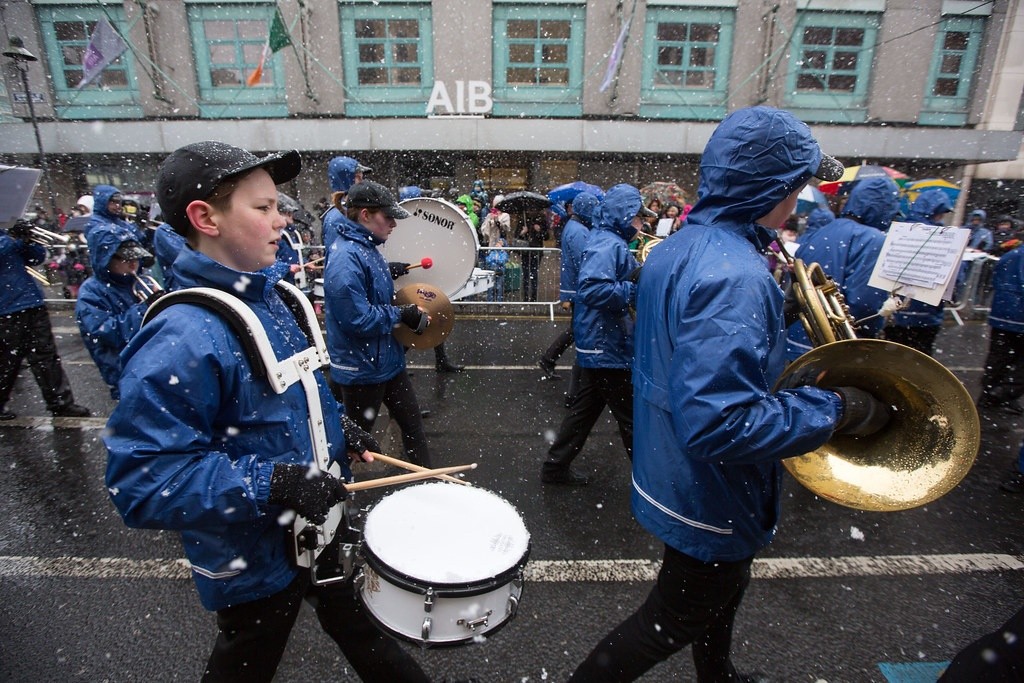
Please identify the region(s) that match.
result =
[894,210,907,218]
[155,141,302,224]
[343,181,411,219]
[110,193,121,201]
[813,152,845,182]
[945,207,956,214]
[637,196,658,218]
[115,238,153,262]
[275,191,300,212]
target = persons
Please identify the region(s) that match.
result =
[935,607,1024,683]
[277,155,373,286]
[0,230,91,421]
[398,177,575,313]
[389,342,465,419]
[35,184,186,299]
[102,140,436,683]
[538,184,694,483]
[571,107,893,683]
[322,182,432,469]
[77,225,167,399]
[770,164,1024,475]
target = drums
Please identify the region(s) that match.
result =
[473,270,496,288]
[448,276,477,303]
[472,273,488,294]
[375,197,480,299]
[312,278,324,300]
[356,480,531,650]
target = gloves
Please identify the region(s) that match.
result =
[266,463,349,526]
[7,221,34,239]
[388,262,411,281]
[830,387,889,439]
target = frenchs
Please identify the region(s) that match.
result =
[771,229,981,512]
[627,231,665,323]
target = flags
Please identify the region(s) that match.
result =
[247,11,290,85]
[600,17,631,91]
[78,16,129,86]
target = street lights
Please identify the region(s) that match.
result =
[0,35,60,229]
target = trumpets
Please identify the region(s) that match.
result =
[4,222,80,251]
[129,268,162,302]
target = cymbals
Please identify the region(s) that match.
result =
[392,283,454,350]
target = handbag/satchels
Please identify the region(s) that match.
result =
[511,234,529,252]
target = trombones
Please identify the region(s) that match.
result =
[25,265,55,286]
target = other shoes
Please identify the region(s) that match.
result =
[541,470,589,486]
[538,359,563,379]
[0,411,17,421]
[53,403,90,418]
[420,410,430,417]
[434,359,464,371]
[1000,401,1024,415]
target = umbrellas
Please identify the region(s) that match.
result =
[816,159,913,196]
[495,192,553,227]
[902,178,961,202]
[547,181,605,201]
[639,181,688,203]
[790,184,828,216]
